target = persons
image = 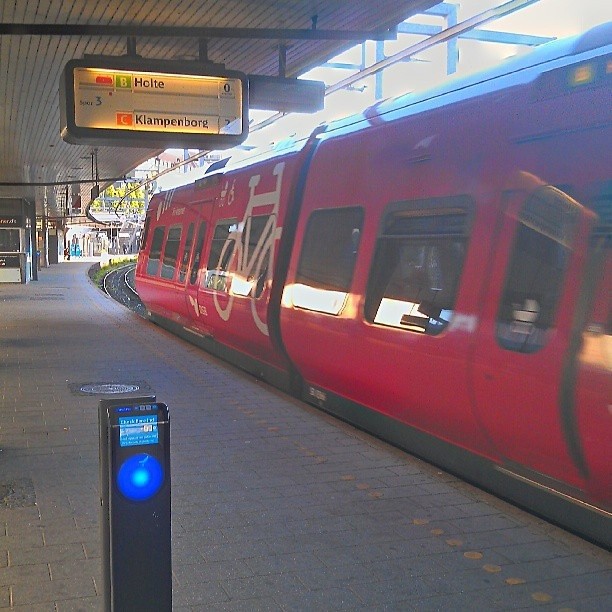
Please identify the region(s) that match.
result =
[65,245,72,261]
[122,242,127,254]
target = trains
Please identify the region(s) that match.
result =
[133,20,611,552]
